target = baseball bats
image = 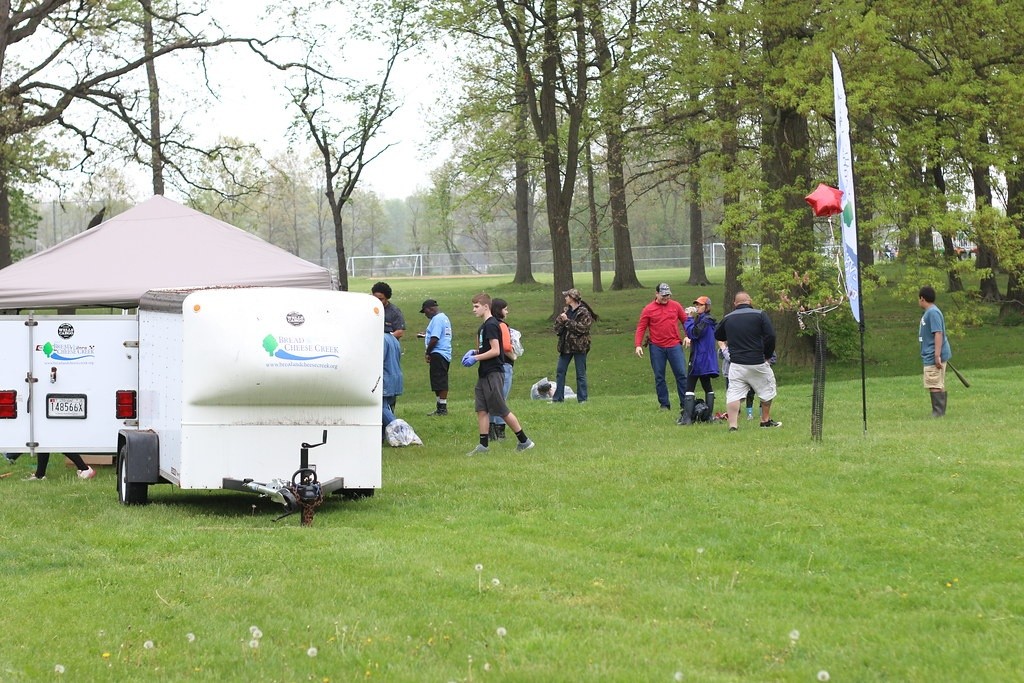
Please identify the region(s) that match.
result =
[947,361,970,388]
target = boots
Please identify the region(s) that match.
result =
[676,391,695,425]
[705,391,716,422]
[930,391,948,417]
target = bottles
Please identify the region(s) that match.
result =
[685,307,697,313]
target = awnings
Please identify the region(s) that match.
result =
[0,194,332,314]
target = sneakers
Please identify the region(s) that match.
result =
[760,417,783,428]
[746,413,754,421]
[466,444,489,456]
[514,438,535,452]
[77,465,95,479]
[427,406,448,416]
[728,427,736,432]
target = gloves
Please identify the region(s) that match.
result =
[461,350,477,362]
[462,356,477,368]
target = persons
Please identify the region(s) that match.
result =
[531,377,577,400]
[933,239,978,261]
[547,288,598,405]
[419,299,453,416]
[382,318,404,437]
[488,298,518,443]
[27,452,95,481]
[714,292,782,434]
[461,293,535,457]
[372,282,406,441]
[814,233,899,268]
[635,283,691,415]
[674,296,720,426]
[717,341,777,421]
[2,453,24,464]
[918,285,952,417]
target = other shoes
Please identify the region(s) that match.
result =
[27,473,46,481]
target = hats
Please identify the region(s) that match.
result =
[562,288,582,302]
[419,299,438,314]
[693,295,711,304]
[656,282,672,296]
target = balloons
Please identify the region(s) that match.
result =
[805,182,844,216]
